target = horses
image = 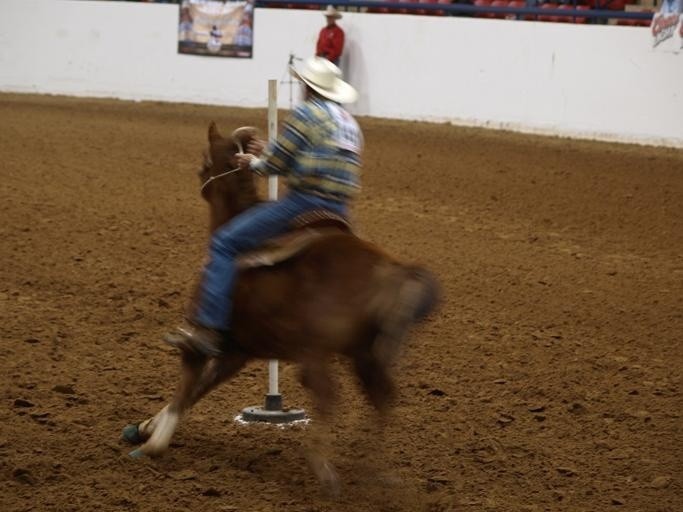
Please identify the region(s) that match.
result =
[122,119,441,505]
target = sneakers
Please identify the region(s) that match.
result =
[161,318,235,360]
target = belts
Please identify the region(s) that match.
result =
[293,185,347,204]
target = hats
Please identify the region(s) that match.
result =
[286,55,360,107]
[320,4,343,20]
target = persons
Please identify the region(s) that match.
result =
[306,2,347,67]
[160,52,366,364]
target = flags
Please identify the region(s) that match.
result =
[174,1,255,60]
